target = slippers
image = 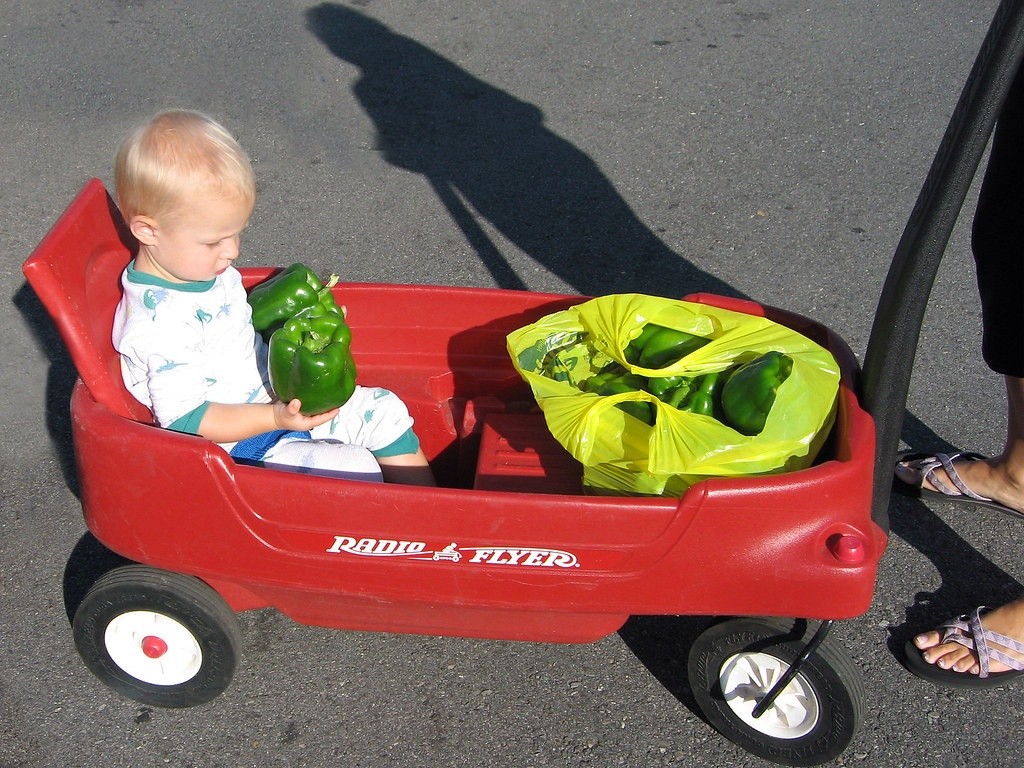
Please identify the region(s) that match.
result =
[904,606,1024,687]
[891,450,1024,519]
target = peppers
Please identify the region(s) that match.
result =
[247,263,345,332]
[266,316,357,417]
[585,325,795,436]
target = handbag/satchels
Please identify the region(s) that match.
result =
[506,293,841,497]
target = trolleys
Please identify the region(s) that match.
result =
[22,1,1023,767]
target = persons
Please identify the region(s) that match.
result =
[889,52,1024,692]
[111,108,437,488]
